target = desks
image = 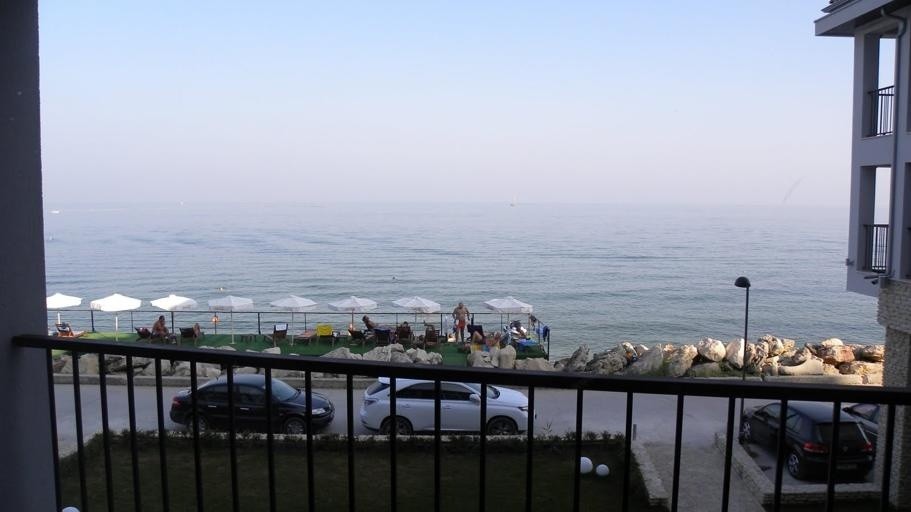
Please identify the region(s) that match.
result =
[240,333,258,344]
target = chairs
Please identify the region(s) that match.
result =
[265,323,495,350]
[133,326,205,345]
[55,323,84,338]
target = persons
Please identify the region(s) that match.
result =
[353,314,378,344]
[150,315,177,345]
[510,321,528,349]
[191,322,201,346]
[453,302,471,347]
[469,330,503,347]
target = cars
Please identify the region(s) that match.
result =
[359,374,537,436]
[169,372,336,436]
[741,396,876,484]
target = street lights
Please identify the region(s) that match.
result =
[733,275,752,442]
